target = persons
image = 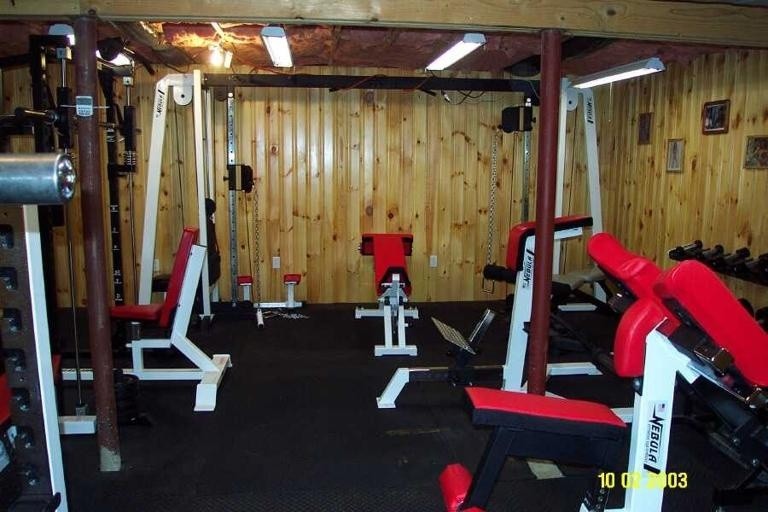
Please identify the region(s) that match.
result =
[195,198,222,313]
[715,107,724,128]
[746,140,768,169]
[705,109,712,129]
[670,142,679,169]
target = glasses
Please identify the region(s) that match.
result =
[260,26,294,68]
[568,57,667,89]
[425,30,486,72]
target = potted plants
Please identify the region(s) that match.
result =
[701,99,730,134]
[742,134,768,170]
[665,138,686,173]
[637,112,655,145]
